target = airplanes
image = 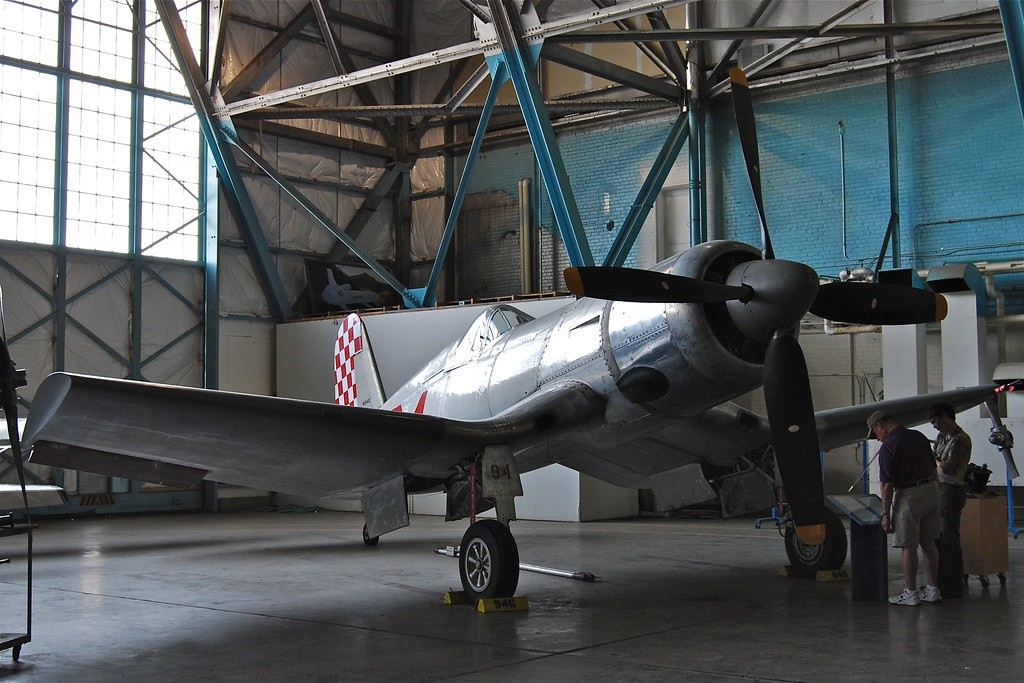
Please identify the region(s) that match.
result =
[19,238,1018,606]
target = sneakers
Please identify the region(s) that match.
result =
[889,588,920,606]
[918,587,942,602]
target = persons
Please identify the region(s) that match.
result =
[926,401,972,597]
[865,410,943,606]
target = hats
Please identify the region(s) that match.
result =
[866,410,888,440]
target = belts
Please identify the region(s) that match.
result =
[899,477,935,489]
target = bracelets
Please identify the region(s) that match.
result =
[883,512,887,515]
